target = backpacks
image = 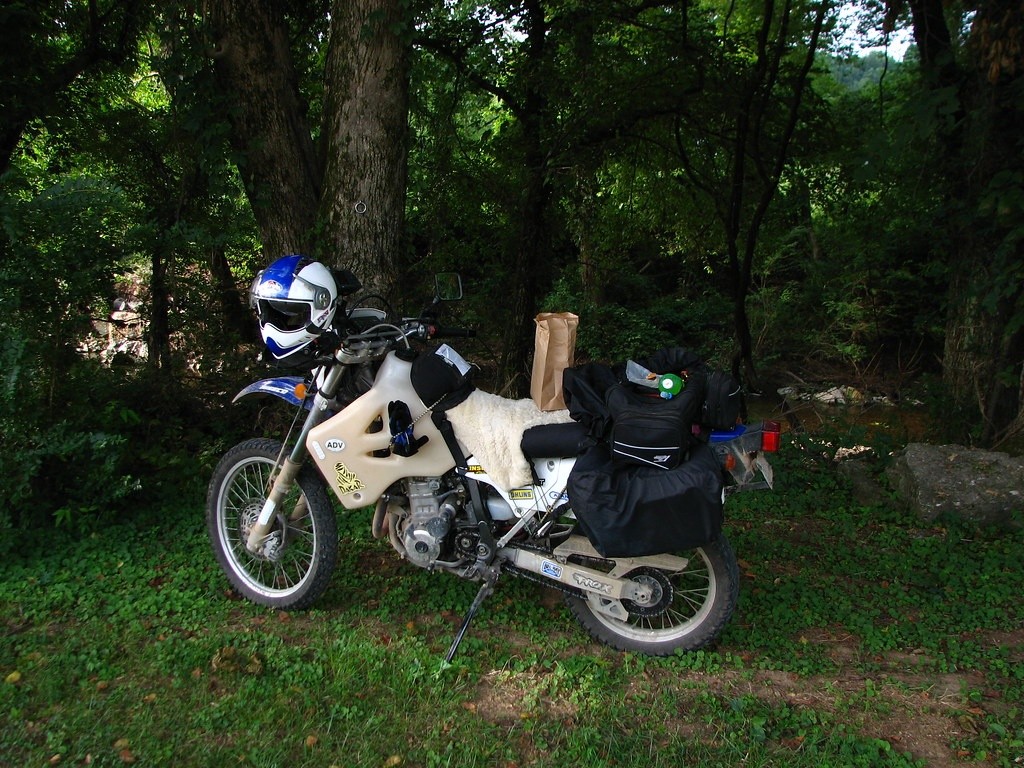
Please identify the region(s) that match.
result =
[702,366,748,432]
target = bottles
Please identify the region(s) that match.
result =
[658,374,684,395]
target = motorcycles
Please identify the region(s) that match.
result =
[204,268,780,668]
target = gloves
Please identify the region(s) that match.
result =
[387,400,429,457]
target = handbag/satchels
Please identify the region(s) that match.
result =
[604,380,698,470]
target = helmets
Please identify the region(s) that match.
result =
[254,254,338,359]
